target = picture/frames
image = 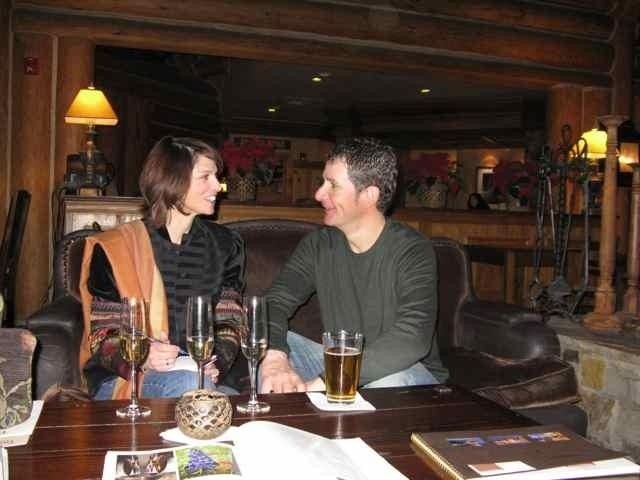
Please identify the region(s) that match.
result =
[475,166,531,213]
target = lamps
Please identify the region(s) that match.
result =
[63,81,119,196]
[569,119,622,182]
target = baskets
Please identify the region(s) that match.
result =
[225,174,256,202]
[416,184,444,208]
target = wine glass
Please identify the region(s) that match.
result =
[116,296,152,418]
[236,295,271,413]
[185,296,214,388]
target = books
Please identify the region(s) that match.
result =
[410,423,640,480]
[102,437,409,480]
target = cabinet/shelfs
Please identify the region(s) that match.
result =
[431,223,622,312]
[64,198,145,237]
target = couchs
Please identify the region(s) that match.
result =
[25,219,587,438]
[0,323,37,429]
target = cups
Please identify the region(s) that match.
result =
[322,329,363,404]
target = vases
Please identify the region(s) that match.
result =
[505,196,521,212]
[421,190,443,210]
[234,179,255,202]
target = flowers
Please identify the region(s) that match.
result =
[491,158,536,203]
[217,135,288,187]
[406,151,460,193]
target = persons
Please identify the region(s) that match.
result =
[248,137,450,394]
[79,135,249,401]
